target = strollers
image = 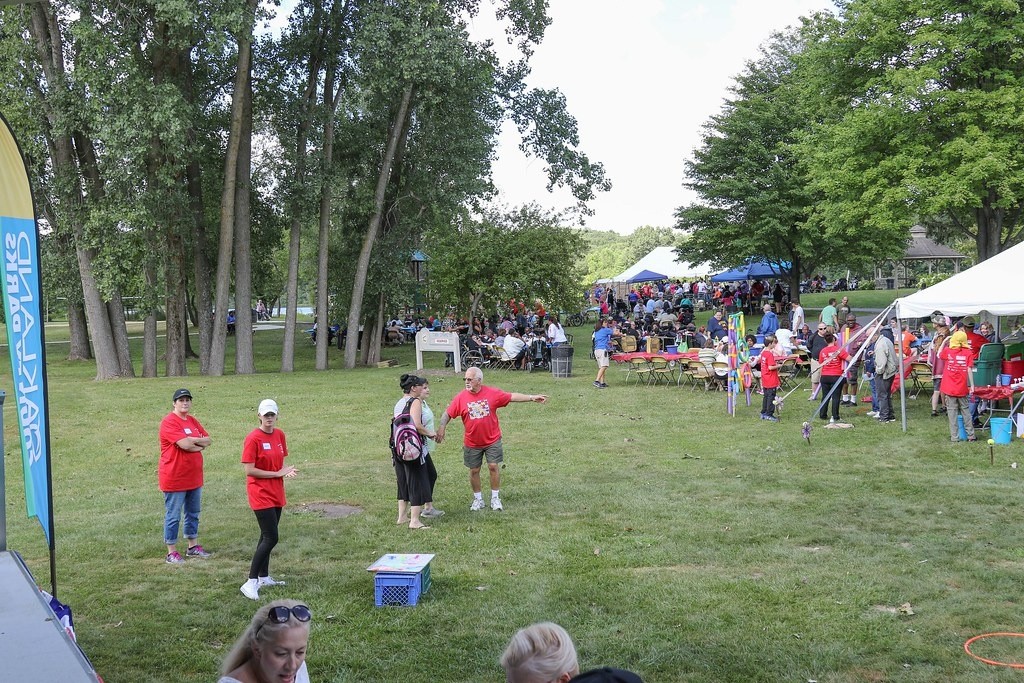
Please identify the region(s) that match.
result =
[832,278,847,291]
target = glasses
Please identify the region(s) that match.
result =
[818,327,826,331]
[256,605,312,636]
[463,378,477,382]
[264,413,275,417]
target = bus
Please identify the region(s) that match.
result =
[104,295,167,320]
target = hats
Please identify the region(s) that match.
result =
[789,298,798,303]
[173,388,192,402]
[258,398,279,416]
[931,315,946,326]
[761,304,771,311]
[962,316,975,328]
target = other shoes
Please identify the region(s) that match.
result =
[930,407,991,443]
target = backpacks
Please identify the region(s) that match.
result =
[389,397,424,462]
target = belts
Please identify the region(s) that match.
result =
[813,357,819,361]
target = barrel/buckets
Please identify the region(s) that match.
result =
[665,345,678,354]
[957,414,969,442]
[748,347,761,356]
[990,417,1012,445]
[754,334,765,344]
[1001,374,1012,386]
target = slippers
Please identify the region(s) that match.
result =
[397,518,410,524]
[409,524,429,530]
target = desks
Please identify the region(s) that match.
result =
[307,327,335,332]
[910,338,932,351]
[891,354,928,393]
[968,385,1024,433]
[611,351,701,384]
[402,327,435,344]
[756,356,803,364]
[651,336,676,351]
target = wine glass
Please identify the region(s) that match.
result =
[1014,376,1024,386]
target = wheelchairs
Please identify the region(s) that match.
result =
[461,346,491,370]
[567,313,585,327]
[526,340,552,373]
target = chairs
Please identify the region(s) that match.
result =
[388,331,401,348]
[750,349,810,395]
[485,345,517,373]
[301,330,314,344]
[904,363,933,400]
[631,347,728,394]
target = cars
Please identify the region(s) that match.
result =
[585,307,601,316]
[544,303,560,320]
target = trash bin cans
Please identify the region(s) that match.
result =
[551,344,574,377]
[252,308,257,323]
[886,279,894,290]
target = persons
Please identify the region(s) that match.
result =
[240,399,300,600]
[218,599,313,683]
[416,378,446,518]
[388,374,436,530]
[584,272,1024,443]
[499,622,580,683]
[435,367,549,513]
[213,299,270,336]
[159,388,212,564]
[311,298,568,370]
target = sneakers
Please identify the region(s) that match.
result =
[421,508,445,518]
[593,380,604,388]
[240,580,259,601]
[469,497,485,511]
[490,499,503,512]
[166,551,187,564]
[185,545,211,557]
[259,576,285,586]
[601,383,610,388]
[760,394,895,423]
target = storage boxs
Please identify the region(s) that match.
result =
[968,343,1024,386]
[647,337,659,353]
[374,562,432,607]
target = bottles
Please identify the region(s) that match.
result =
[996,374,1000,388]
[659,348,663,355]
[715,336,718,344]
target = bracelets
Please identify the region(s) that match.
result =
[530,395,532,401]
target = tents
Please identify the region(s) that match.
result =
[598,247,733,282]
[709,255,790,316]
[896,241,1024,434]
[626,270,668,291]
